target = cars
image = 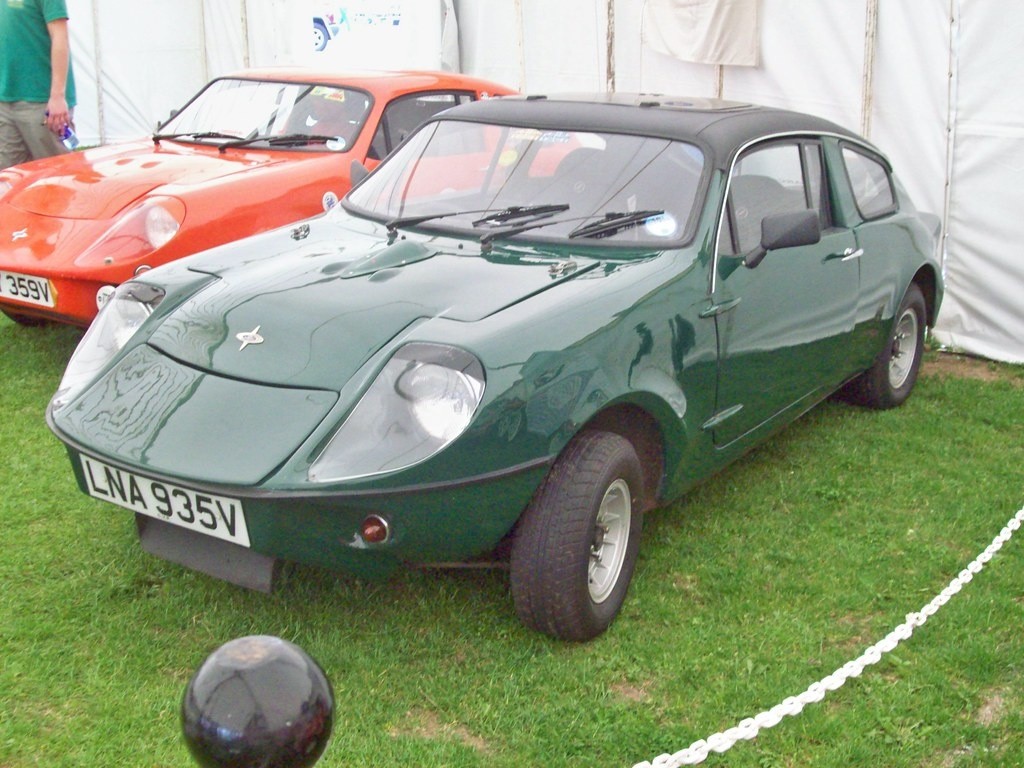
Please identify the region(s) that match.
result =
[0,66,582,332]
[40,85,949,642]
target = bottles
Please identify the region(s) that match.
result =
[45,111,79,149]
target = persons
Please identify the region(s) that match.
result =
[0,0,77,173]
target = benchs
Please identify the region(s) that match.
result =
[360,100,461,161]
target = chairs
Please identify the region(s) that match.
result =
[542,145,631,230]
[722,175,791,252]
[300,94,357,146]
